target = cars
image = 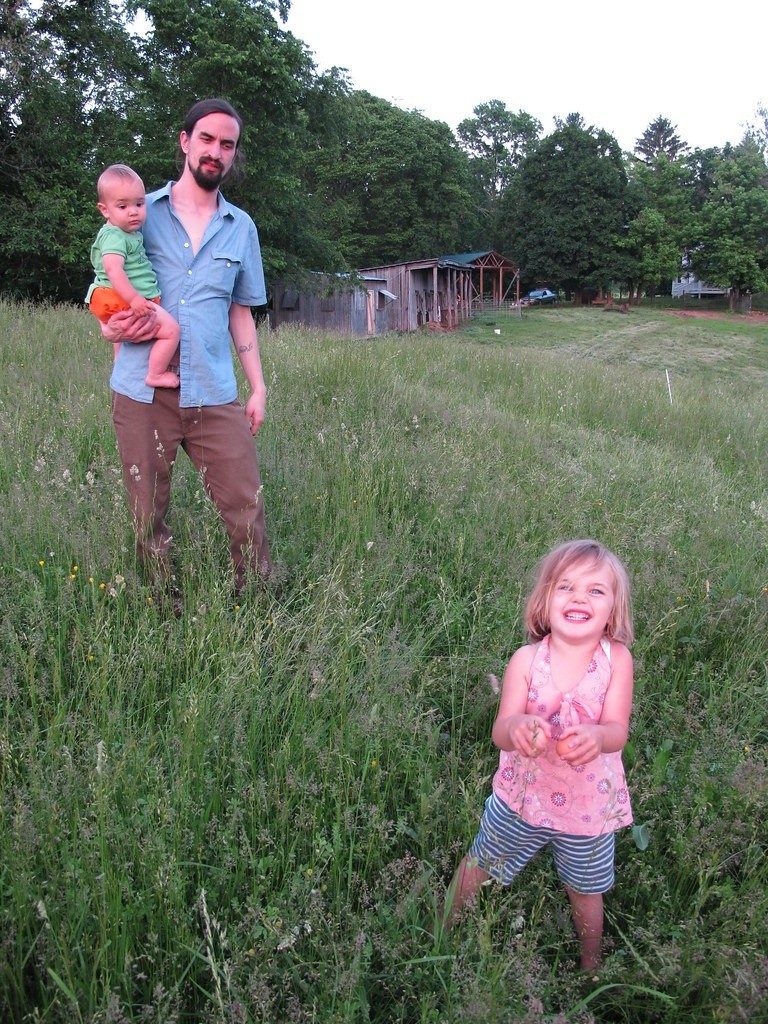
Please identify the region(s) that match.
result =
[518,288,558,306]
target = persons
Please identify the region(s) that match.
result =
[84,164,179,388]
[99,100,274,614]
[439,539,636,970]
